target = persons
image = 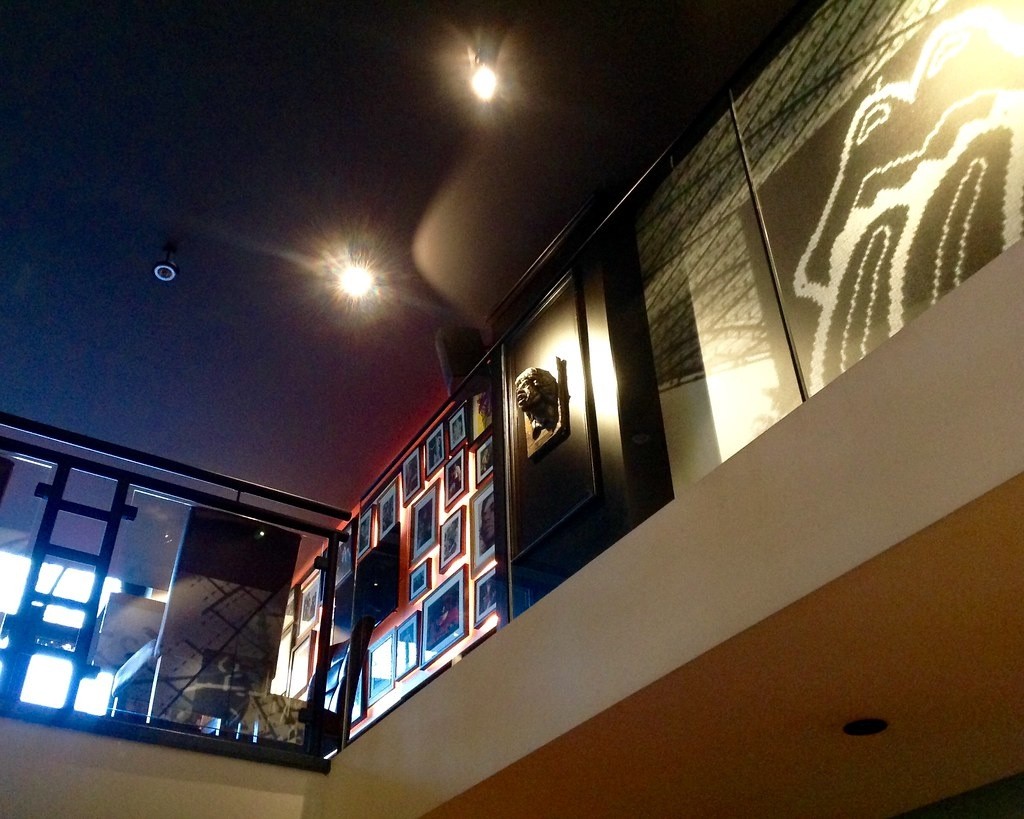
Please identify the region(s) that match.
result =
[514,367,559,427]
[479,492,495,553]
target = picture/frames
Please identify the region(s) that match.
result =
[266,390,501,730]
